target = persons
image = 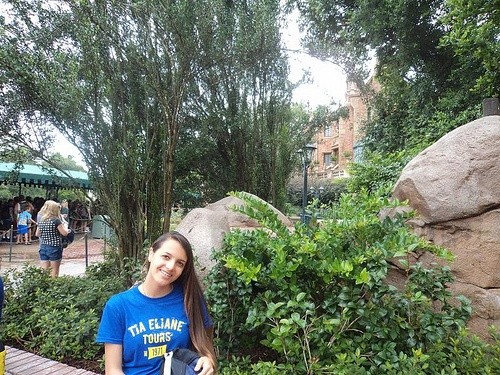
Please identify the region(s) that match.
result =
[0,195,107,245]
[35,200,68,278]
[96,232,215,375]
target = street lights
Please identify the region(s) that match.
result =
[297,141,318,228]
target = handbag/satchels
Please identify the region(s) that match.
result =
[160,350,212,375]
[58,226,78,251]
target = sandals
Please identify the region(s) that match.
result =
[20,240,34,246]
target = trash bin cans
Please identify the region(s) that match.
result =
[91,214,110,239]
